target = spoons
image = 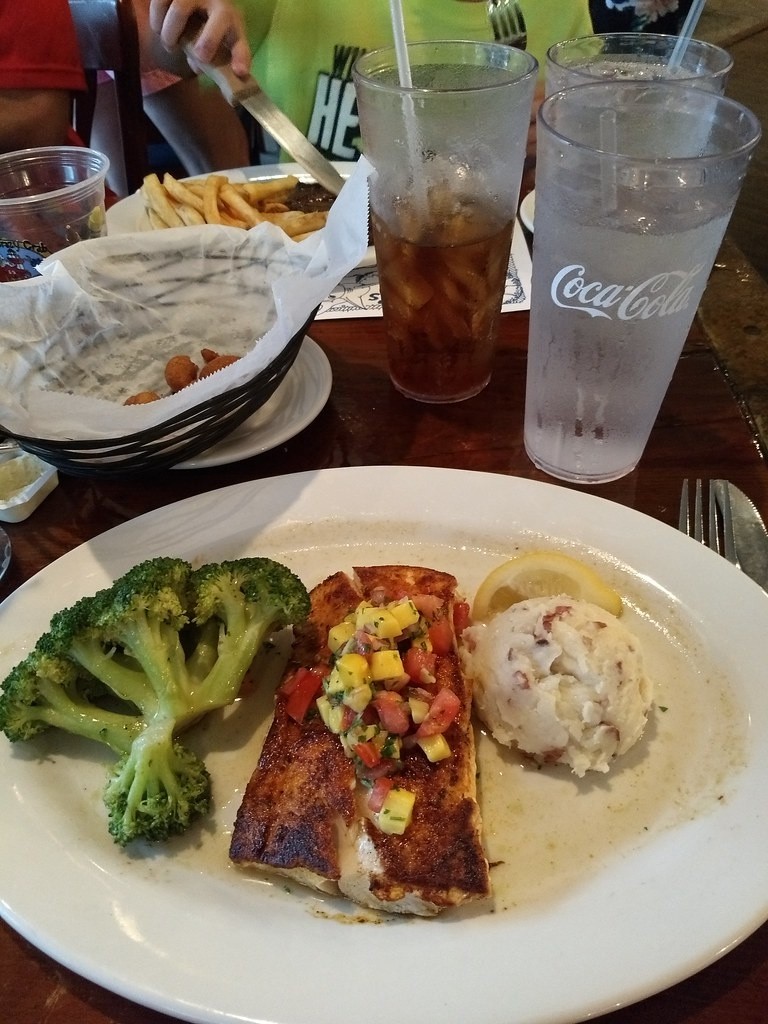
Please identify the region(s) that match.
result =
[711,477,768,595]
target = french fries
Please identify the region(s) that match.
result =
[142,172,327,235]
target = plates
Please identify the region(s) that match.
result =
[0,464,768,1024]
[163,334,334,473]
[100,158,379,269]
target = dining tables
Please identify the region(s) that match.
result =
[0,153,766,1023]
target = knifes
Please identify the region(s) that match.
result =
[176,10,347,200]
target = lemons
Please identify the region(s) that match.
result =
[470,550,624,625]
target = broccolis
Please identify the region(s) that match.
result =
[1,556,312,848]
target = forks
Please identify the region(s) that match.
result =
[672,479,737,573]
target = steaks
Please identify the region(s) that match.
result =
[287,181,374,245]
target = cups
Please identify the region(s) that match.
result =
[523,77,762,486]
[0,144,110,284]
[350,37,539,403]
[542,33,734,117]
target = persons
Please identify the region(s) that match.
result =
[87,0,250,202]
[0,0,118,250]
[146,0,603,165]
